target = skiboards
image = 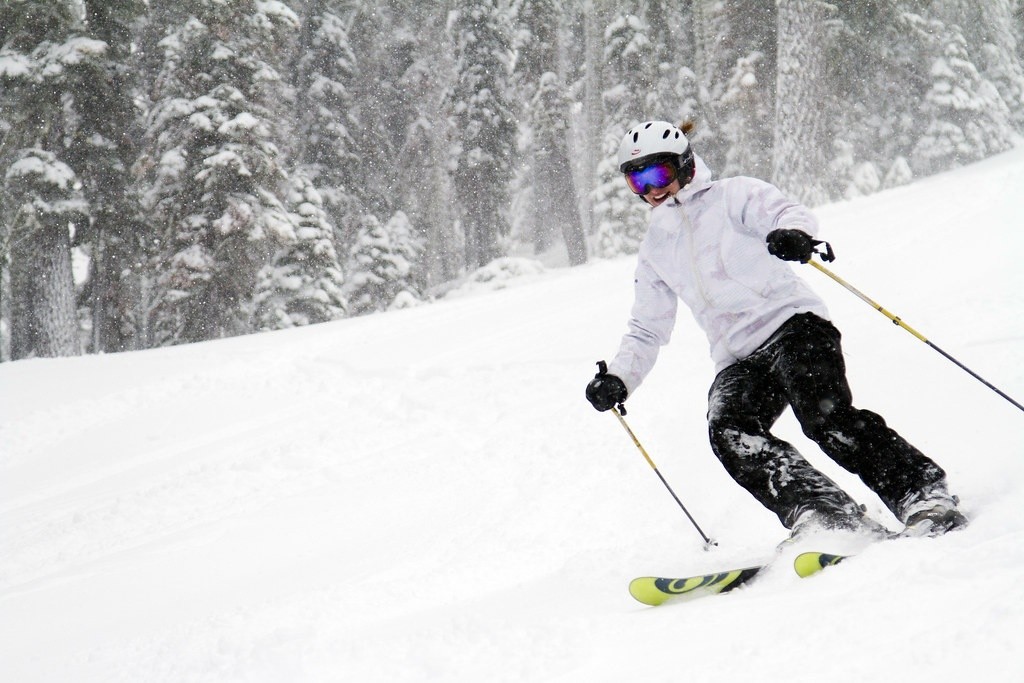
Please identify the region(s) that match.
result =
[627,551,845,608]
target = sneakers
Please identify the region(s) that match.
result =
[904,479,957,531]
[779,511,857,556]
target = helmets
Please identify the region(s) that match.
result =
[619,122,692,183]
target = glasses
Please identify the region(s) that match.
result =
[625,153,694,195]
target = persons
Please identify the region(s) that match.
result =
[581,116,970,548]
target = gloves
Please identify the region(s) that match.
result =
[584,375,627,412]
[765,229,812,266]
